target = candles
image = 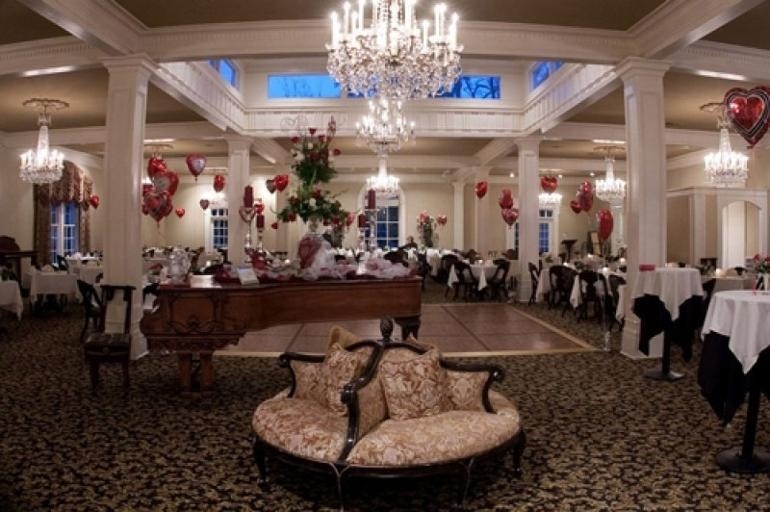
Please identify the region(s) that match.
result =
[366,189,377,209]
[242,185,253,208]
[255,214,264,228]
[358,213,366,228]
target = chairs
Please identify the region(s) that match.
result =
[0,228,224,390]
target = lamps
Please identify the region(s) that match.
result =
[355,93,418,198]
[697,99,751,190]
[15,97,73,188]
[538,167,565,205]
[592,145,628,203]
[318,0,466,111]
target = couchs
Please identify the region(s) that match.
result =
[249,324,529,510]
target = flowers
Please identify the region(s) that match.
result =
[270,120,359,237]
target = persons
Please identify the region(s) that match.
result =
[603,255,626,298]
[398,235,417,249]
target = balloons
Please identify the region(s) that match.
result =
[265,178,276,194]
[569,181,594,213]
[213,175,225,192]
[270,223,278,229]
[501,210,518,227]
[720,85,770,150]
[142,157,178,221]
[176,208,186,218]
[200,199,209,211]
[253,203,265,214]
[186,153,207,180]
[89,194,100,210]
[595,209,613,240]
[275,175,288,192]
[540,175,559,196]
[474,181,489,199]
[435,214,447,224]
[499,189,514,209]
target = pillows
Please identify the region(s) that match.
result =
[405,329,445,362]
[376,350,452,424]
[315,339,364,419]
[324,325,361,354]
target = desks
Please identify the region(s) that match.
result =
[619,249,769,479]
